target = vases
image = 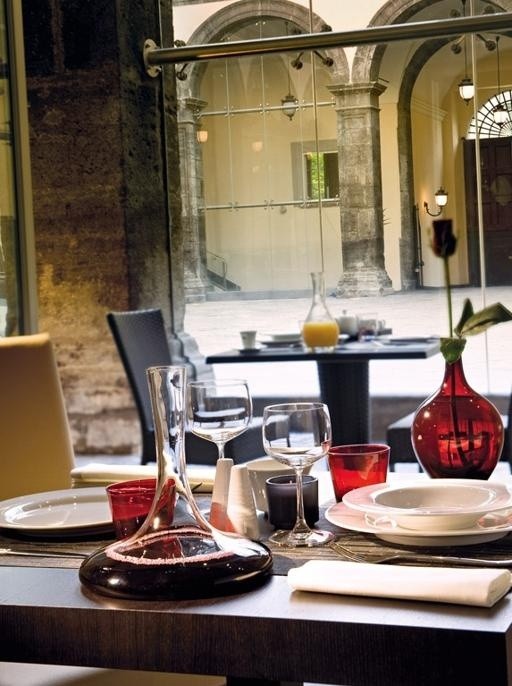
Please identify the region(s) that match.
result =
[410,359,505,481]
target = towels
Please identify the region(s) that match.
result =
[287,558,512,608]
[69,465,214,494]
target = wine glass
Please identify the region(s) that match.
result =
[183,379,253,456]
[259,401,338,548]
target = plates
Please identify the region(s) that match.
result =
[258,340,301,347]
[341,478,511,529]
[325,502,510,547]
[263,325,302,340]
[232,344,268,353]
[384,333,432,342]
[0,486,113,537]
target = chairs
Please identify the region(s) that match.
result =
[0,332,77,544]
[387,394,512,474]
[106,308,291,465]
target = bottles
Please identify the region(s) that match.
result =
[334,309,361,336]
[302,269,340,352]
[78,363,273,604]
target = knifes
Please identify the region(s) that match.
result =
[0,547,89,559]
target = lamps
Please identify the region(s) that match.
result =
[423,186,448,216]
[281,22,296,120]
[458,0,474,105]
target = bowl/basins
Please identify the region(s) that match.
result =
[246,459,313,510]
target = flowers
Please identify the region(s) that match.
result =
[431,219,512,476]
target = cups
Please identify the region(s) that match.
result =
[239,330,257,349]
[264,474,319,531]
[328,446,390,500]
[106,478,178,545]
[358,311,386,335]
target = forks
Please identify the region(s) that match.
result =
[370,339,409,349]
[329,541,511,568]
